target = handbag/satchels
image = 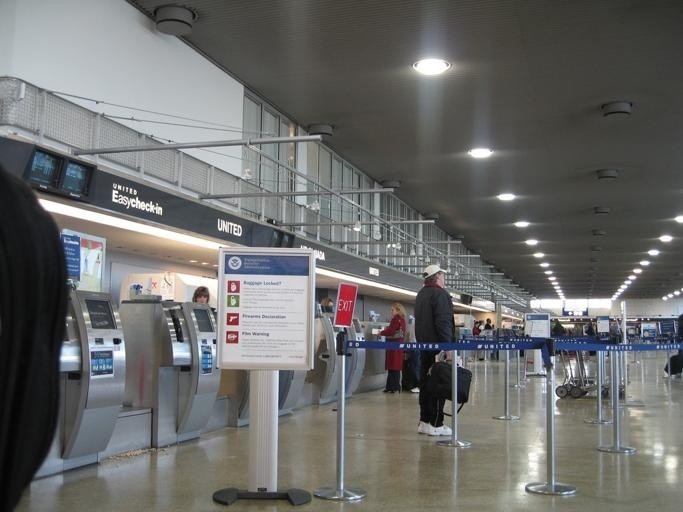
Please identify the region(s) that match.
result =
[386,328,403,339]
[431,361,472,402]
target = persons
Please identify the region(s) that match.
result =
[472,321,480,335]
[585,318,596,356]
[484,318,491,329]
[192,287,209,303]
[551,319,564,337]
[413,265,454,435]
[376,303,405,394]
[320,296,333,306]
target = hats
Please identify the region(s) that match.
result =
[424,265,447,279]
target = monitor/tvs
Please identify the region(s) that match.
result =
[328,316,340,333]
[82,296,117,330]
[252,225,296,249]
[461,294,473,304]
[192,307,215,333]
[23,144,63,196]
[351,318,361,333]
[59,156,96,205]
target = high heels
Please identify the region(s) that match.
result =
[384,390,401,394]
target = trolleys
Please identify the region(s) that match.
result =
[555,349,630,398]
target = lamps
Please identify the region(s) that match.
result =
[240,144,442,269]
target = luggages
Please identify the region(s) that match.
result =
[664,354,682,375]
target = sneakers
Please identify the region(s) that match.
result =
[417,421,452,435]
[410,387,420,393]
